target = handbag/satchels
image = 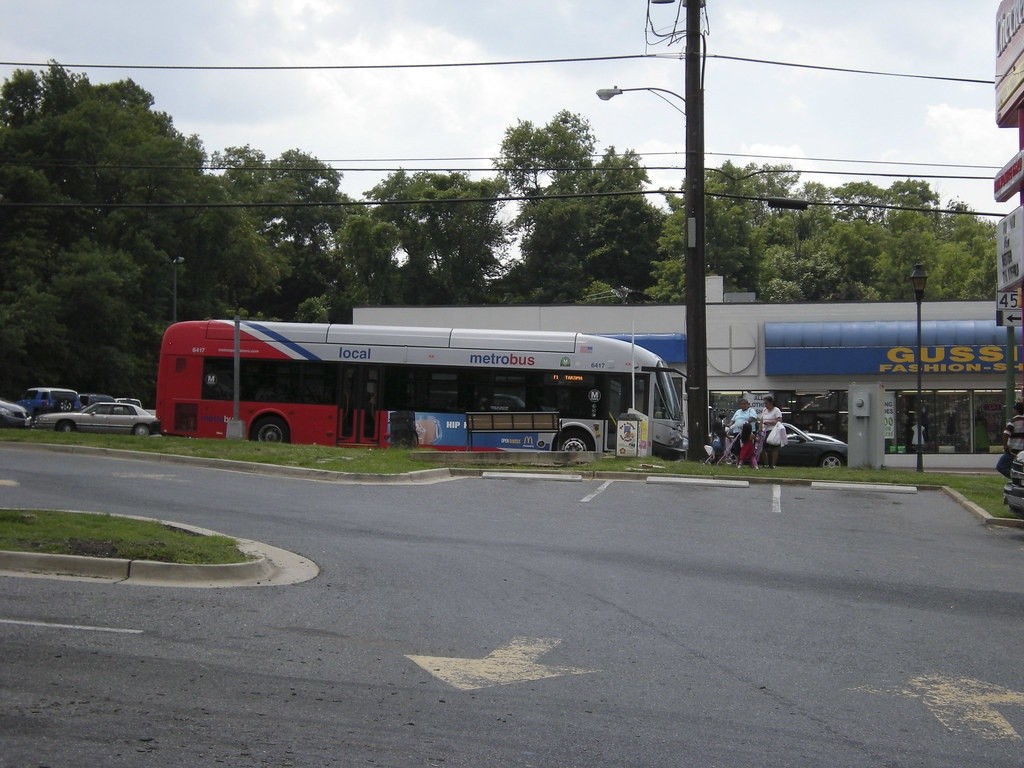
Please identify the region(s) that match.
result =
[766,422,782,447]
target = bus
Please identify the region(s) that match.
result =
[153,318,683,465]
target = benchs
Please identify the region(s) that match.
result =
[465,410,562,451]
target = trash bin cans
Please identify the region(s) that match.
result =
[617,413,642,458]
[390,411,419,449]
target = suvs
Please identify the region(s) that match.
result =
[15,387,80,419]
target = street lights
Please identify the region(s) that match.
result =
[595,87,712,463]
[172,254,186,324]
[907,264,929,468]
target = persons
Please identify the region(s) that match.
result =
[705,394,789,469]
[1002,403,1024,479]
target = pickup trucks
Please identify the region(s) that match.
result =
[97,398,156,418]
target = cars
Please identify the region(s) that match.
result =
[707,424,848,468]
[784,433,845,443]
[78,393,115,411]
[34,401,160,437]
[0,399,32,429]
